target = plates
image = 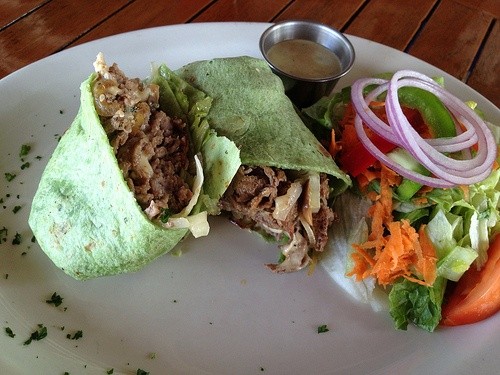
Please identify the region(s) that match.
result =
[1,24,500,375]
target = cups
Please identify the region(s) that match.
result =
[259,20,356,107]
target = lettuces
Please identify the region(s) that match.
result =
[301,85,500,333]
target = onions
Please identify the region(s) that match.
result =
[350,69,497,188]
